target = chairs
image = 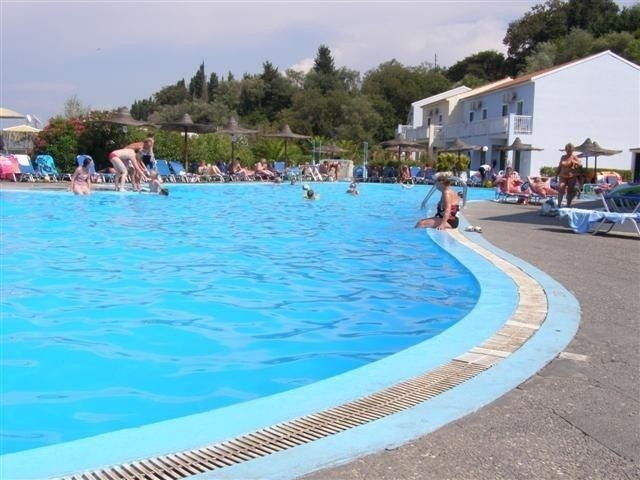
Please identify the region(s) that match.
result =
[354,165,493,185]
[14,153,325,184]
[496,170,559,204]
[556,191,640,238]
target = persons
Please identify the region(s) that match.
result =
[71,158,93,194]
[371,163,583,206]
[346,185,359,196]
[198,141,357,183]
[301,183,321,200]
[414,171,460,230]
[554,143,582,205]
[108,138,163,192]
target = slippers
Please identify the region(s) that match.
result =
[464,225,483,233]
